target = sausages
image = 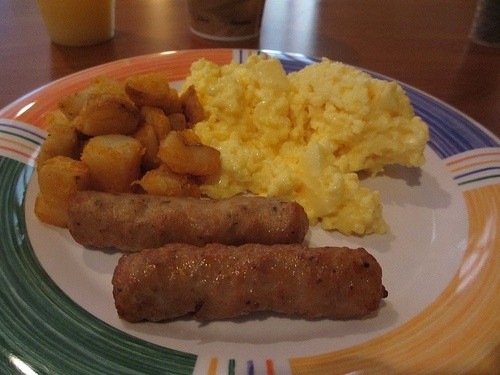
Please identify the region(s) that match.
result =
[66,185,311,252]
[110,243,388,323]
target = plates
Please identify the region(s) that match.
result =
[1,50,499,375]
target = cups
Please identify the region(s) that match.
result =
[469,0,500,47]
[187,0,264,40]
[36,1,115,46]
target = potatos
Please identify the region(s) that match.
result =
[33,71,220,229]
